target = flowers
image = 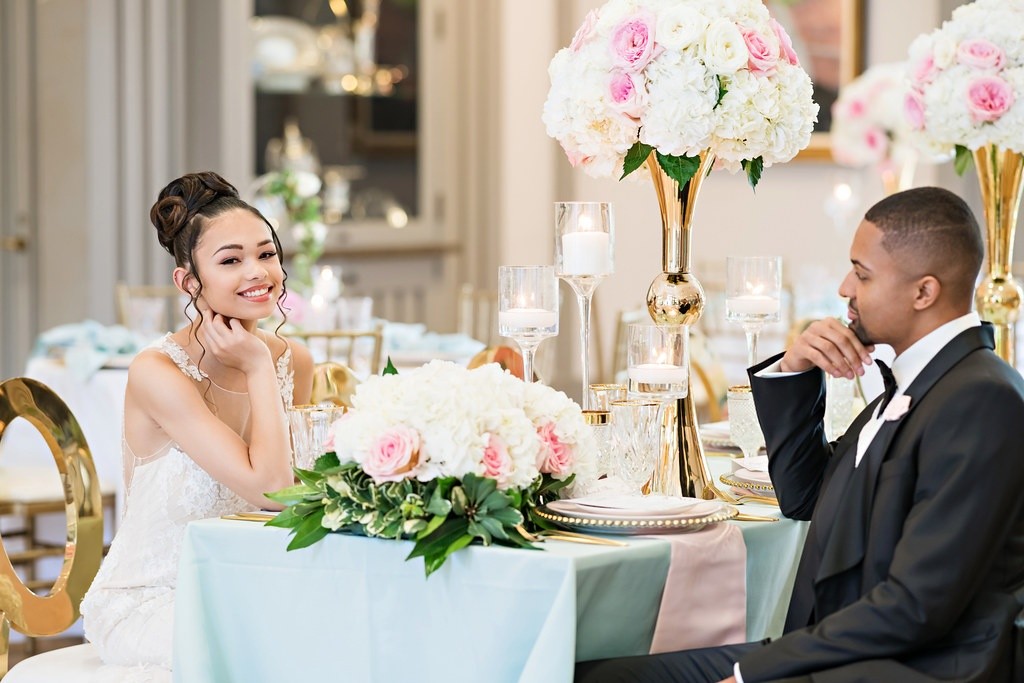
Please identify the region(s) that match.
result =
[893,2,1023,152]
[540,2,820,172]
[829,69,946,168]
[267,361,585,576]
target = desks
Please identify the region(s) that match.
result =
[180,488,813,683]
[1,331,480,517]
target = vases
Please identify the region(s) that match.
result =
[881,156,940,202]
[963,142,1022,368]
[606,142,738,509]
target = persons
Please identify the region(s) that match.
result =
[81,171,315,669]
[574,186,1024,683]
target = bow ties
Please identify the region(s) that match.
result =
[874,358,897,419]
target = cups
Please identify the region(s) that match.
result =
[824,376,854,443]
[581,413,611,481]
[288,404,344,473]
[588,384,627,413]
[308,264,373,333]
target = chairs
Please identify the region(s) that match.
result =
[282,321,384,373]
[469,343,540,383]
[118,285,192,337]
[0,379,152,682]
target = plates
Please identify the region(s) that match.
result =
[254,15,319,92]
[533,496,740,535]
[719,469,776,498]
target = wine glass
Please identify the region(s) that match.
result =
[497,264,561,384]
[726,385,766,467]
[554,201,615,412]
[626,323,690,503]
[607,400,663,507]
[724,256,783,386]
